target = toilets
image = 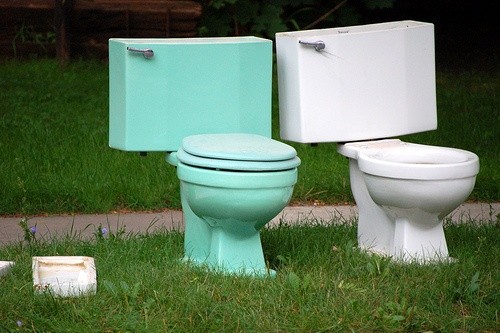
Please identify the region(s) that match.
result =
[108,36,302,278]
[276,19,480,265]
